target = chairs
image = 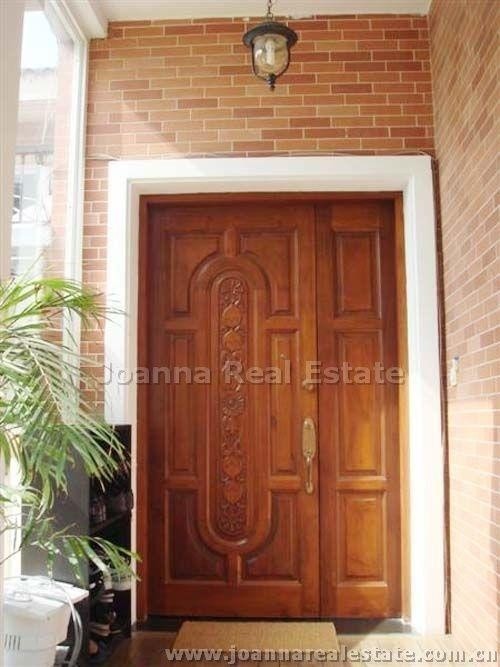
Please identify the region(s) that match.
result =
[241,0,298,90]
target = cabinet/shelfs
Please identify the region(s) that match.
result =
[20,422,133,665]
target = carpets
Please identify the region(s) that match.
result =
[169,619,340,657]
